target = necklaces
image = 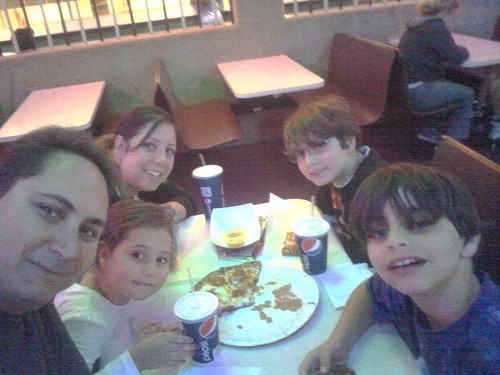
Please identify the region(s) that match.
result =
[113,181,138,202]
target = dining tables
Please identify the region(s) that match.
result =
[216,51,323,114]
[391,27,499,119]
[139,195,425,375]
[0,83,108,145]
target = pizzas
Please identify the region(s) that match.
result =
[281,231,300,256]
[187,261,261,314]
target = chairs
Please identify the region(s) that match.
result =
[290,31,398,131]
[429,138,500,283]
[157,57,246,172]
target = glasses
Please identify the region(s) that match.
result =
[287,135,328,164]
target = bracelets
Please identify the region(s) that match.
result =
[159,203,179,224]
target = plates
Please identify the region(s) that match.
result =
[210,203,261,249]
[188,261,319,347]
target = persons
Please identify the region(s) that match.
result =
[282,92,397,269]
[298,163,499,375]
[93,105,197,229]
[51,198,201,375]
[1,123,124,375]
[472,12,500,144]
[396,0,475,149]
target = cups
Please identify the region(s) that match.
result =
[172,291,219,366]
[292,216,331,276]
[191,165,224,224]
